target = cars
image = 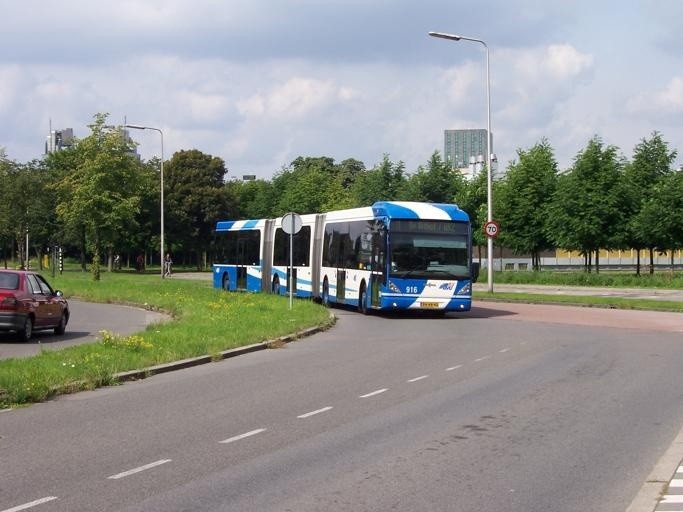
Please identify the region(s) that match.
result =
[2,267,72,343]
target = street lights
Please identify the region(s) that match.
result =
[428,30,495,291]
[122,124,166,277]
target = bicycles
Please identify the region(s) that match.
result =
[165,261,173,278]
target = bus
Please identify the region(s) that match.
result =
[212,200,474,315]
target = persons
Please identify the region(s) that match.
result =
[163,253,173,277]
[390,243,450,270]
[111,251,122,270]
[135,253,146,273]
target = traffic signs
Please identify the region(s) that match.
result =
[483,220,499,237]
